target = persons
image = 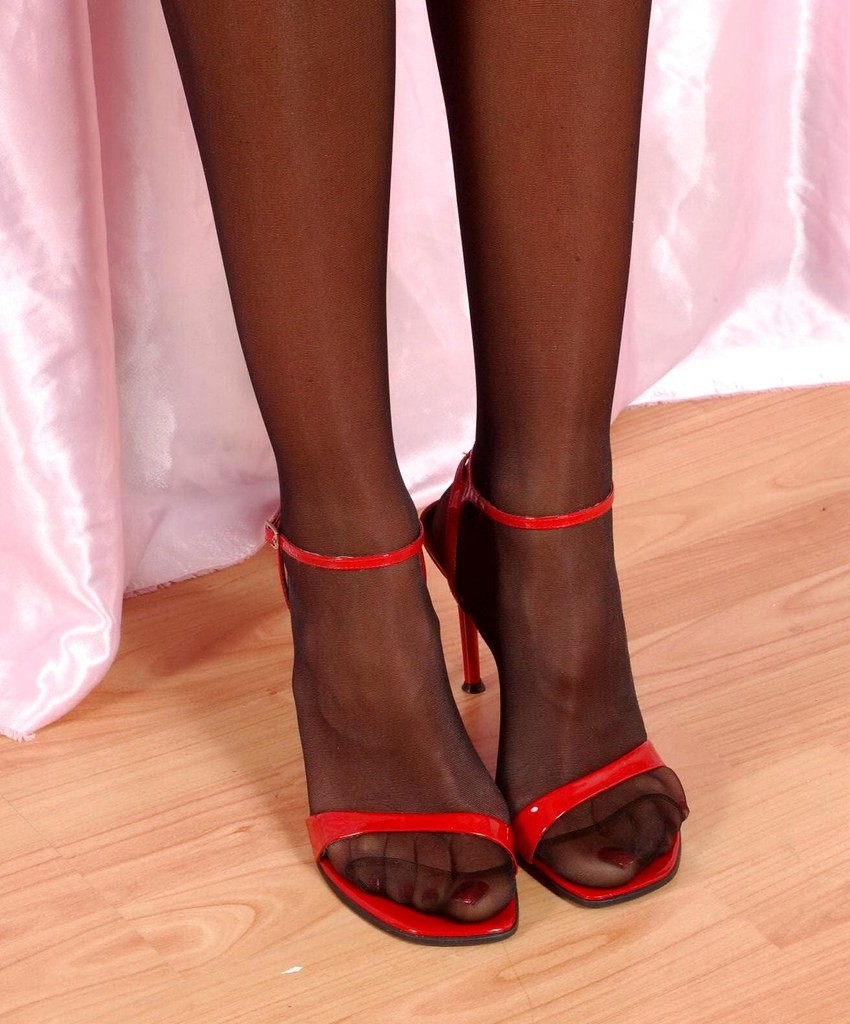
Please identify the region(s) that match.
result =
[171,0,694,949]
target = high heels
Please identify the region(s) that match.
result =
[420,442,682,909]
[263,503,518,946]
